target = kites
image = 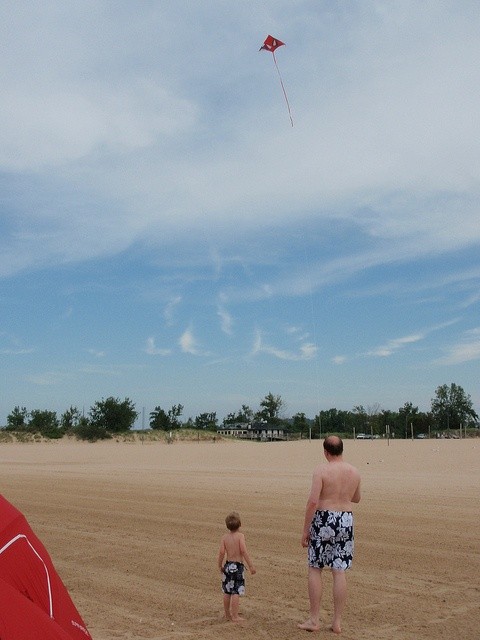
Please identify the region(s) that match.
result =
[258,34,294,127]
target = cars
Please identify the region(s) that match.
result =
[366,434,380,439]
[417,434,426,439]
[356,433,366,439]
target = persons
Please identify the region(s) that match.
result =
[218,512,257,622]
[296,436,362,633]
